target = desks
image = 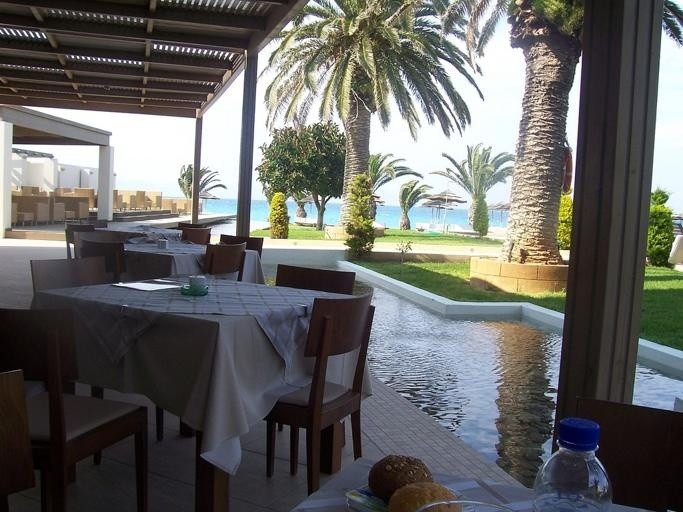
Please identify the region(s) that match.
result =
[33,277,357,511]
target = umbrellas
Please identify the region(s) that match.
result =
[422,189,510,234]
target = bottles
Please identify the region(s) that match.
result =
[531,415,614,512]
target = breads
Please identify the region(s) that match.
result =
[389,481,461,512]
[368,453,432,502]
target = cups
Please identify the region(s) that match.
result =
[188,275,206,287]
[414,499,514,512]
[157,239,169,250]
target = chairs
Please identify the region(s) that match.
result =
[3,370,37,510]
[573,395,681,510]
[263,292,375,497]
[31,256,163,465]
[2,308,149,511]
[65,220,267,285]
[277,264,355,447]
[9,184,196,228]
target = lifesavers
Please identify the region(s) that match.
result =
[561,147,573,194]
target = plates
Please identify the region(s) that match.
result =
[467,500,651,512]
